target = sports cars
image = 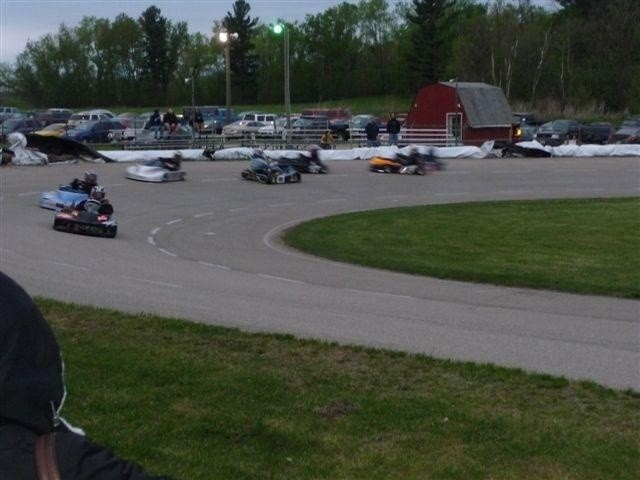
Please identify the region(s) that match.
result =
[276,153,326,173]
[395,155,440,172]
[366,155,425,176]
[240,166,302,185]
[124,159,187,184]
[51,201,119,239]
[41,184,90,209]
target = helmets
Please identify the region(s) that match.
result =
[90,185,106,200]
[84,168,98,182]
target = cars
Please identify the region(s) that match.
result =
[511,111,640,146]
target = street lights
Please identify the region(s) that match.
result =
[273,21,293,147]
[217,27,239,125]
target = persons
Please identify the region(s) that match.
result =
[72,170,98,194]
[193,109,205,131]
[252,149,280,172]
[72,186,113,220]
[149,109,164,139]
[395,148,421,165]
[364,117,379,146]
[164,108,181,134]
[0,272,171,480]
[320,131,333,149]
[307,145,320,162]
[159,151,181,170]
[387,112,400,146]
[422,148,440,162]
